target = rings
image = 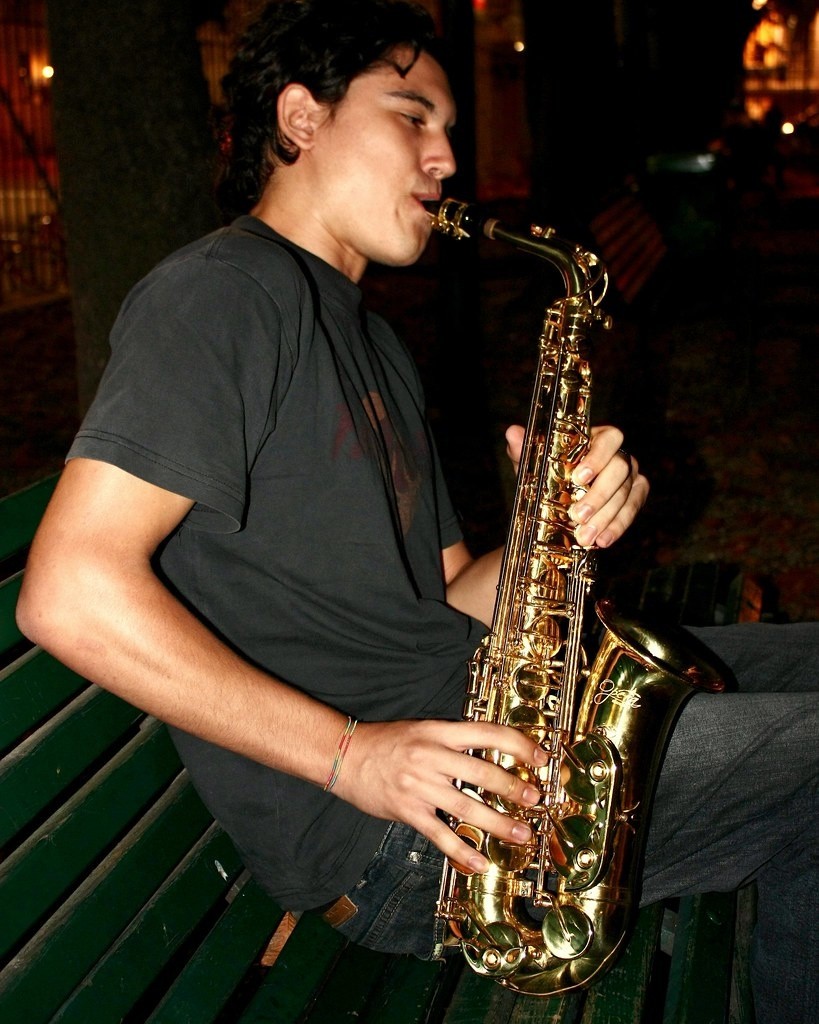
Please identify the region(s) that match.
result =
[618,449,629,458]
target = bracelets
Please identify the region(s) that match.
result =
[323,716,359,795]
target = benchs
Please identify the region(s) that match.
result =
[0,468,755,1024]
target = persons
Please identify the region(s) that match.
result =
[14,0,819,1024]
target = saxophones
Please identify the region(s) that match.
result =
[416,194,725,997]
